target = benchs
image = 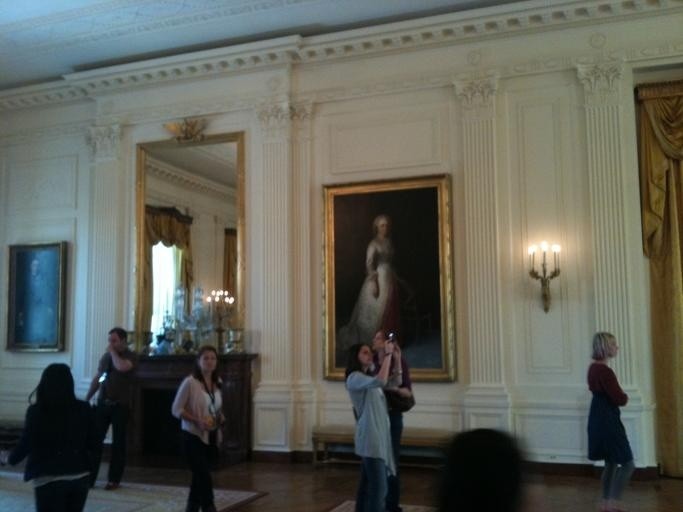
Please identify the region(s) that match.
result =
[311,424,455,467]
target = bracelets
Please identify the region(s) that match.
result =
[382,351,392,358]
[391,367,402,375]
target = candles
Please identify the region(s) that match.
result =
[527,242,560,271]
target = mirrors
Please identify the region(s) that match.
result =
[135,117,245,352]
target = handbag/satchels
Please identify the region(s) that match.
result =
[390,388,415,412]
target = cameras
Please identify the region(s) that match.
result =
[383,329,393,342]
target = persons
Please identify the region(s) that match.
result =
[429,423,526,511]
[363,326,415,512]
[171,343,227,511]
[0,362,101,511]
[328,214,401,356]
[585,330,637,511]
[80,325,136,490]
[343,337,404,511]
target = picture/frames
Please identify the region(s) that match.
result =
[5,241,68,353]
[320,173,456,383]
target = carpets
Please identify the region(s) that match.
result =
[0,450,270,510]
